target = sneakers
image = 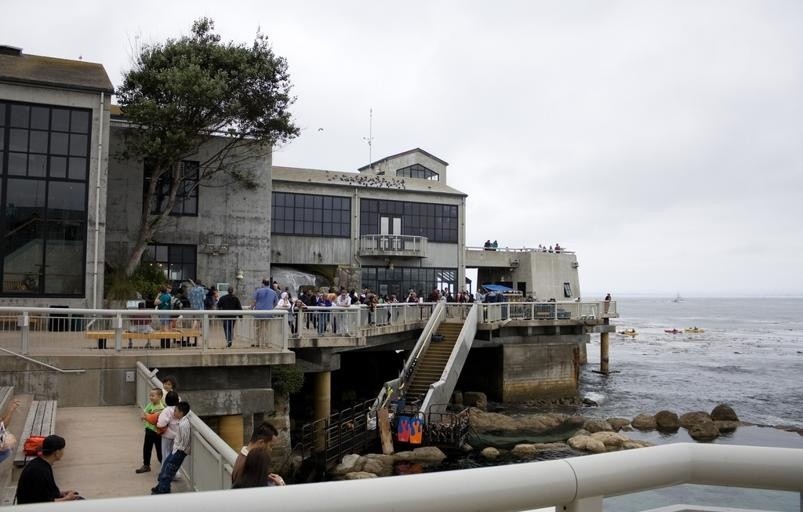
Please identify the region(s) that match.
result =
[151,485,170,494]
[136,465,150,473]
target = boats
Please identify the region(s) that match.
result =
[664,328,705,333]
[619,330,640,337]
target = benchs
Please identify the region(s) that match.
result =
[1,313,43,332]
[87,329,199,349]
[13,399,57,466]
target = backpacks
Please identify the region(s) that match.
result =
[24,434,46,455]
[165,294,182,319]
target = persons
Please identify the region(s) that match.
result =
[128,280,219,348]
[604,293,611,314]
[273,281,485,336]
[136,375,190,494]
[17,435,85,503]
[484,240,498,251]
[216,287,243,347]
[538,244,560,253]
[249,278,278,348]
[575,296,580,302]
[232,421,286,488]
